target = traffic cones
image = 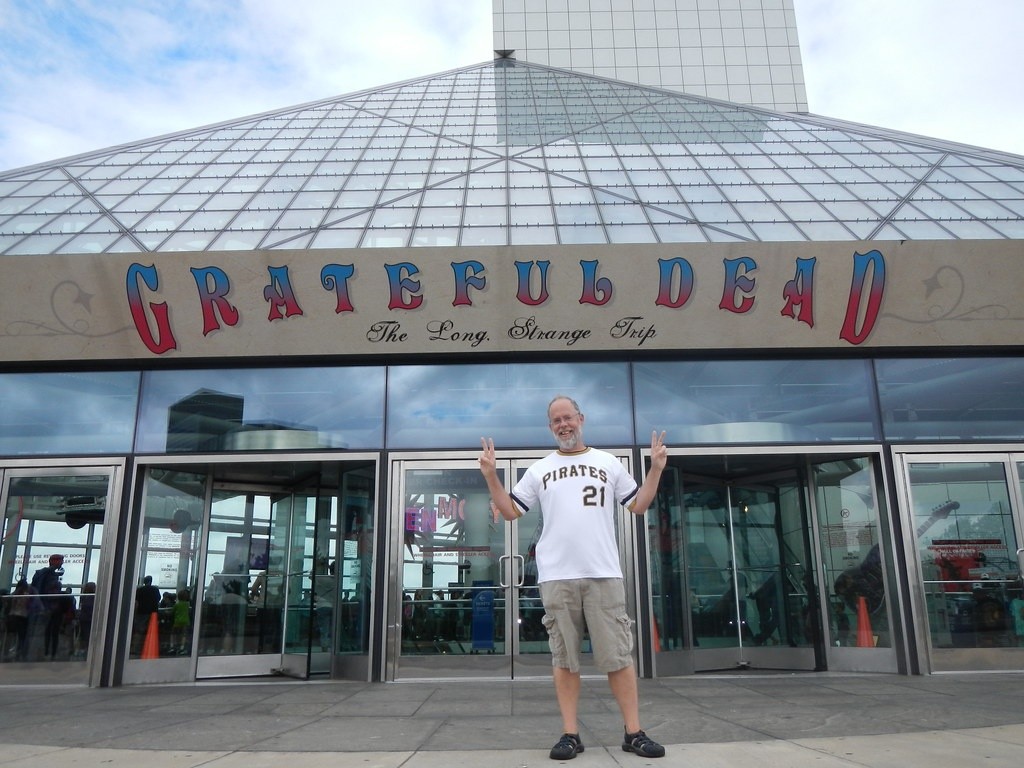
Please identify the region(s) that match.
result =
[140,612,159,659]
[653,617,660,652]
[856,597,875,647]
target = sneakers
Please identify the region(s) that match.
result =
[550,731,584,760]
[622,725,665,758]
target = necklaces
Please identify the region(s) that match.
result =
[557,444,588,453]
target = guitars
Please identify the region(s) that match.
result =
[832,499,961,620]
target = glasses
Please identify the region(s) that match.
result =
[551,413,579,425]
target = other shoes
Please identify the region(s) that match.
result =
[167,647,175,653]
[178,650,185,655]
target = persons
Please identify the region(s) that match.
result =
[726,561,749,637]
[478,396,668,760]
[0,540,539,657]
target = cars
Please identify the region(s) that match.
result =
[924,557,1024,637]
